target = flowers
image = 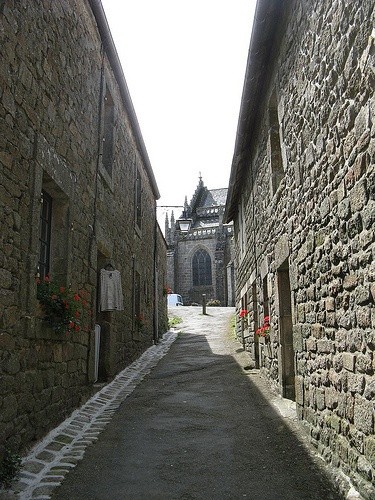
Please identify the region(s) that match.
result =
[256,316,270,337]
[136,315,145,326]
[36,272,87,337]
[239,309,247,322]
[163,287,171,296]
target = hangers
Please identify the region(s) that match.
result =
[100,256,121,276]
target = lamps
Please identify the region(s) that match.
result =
[156,205,193,235]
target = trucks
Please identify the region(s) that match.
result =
[167,293,184,307]
